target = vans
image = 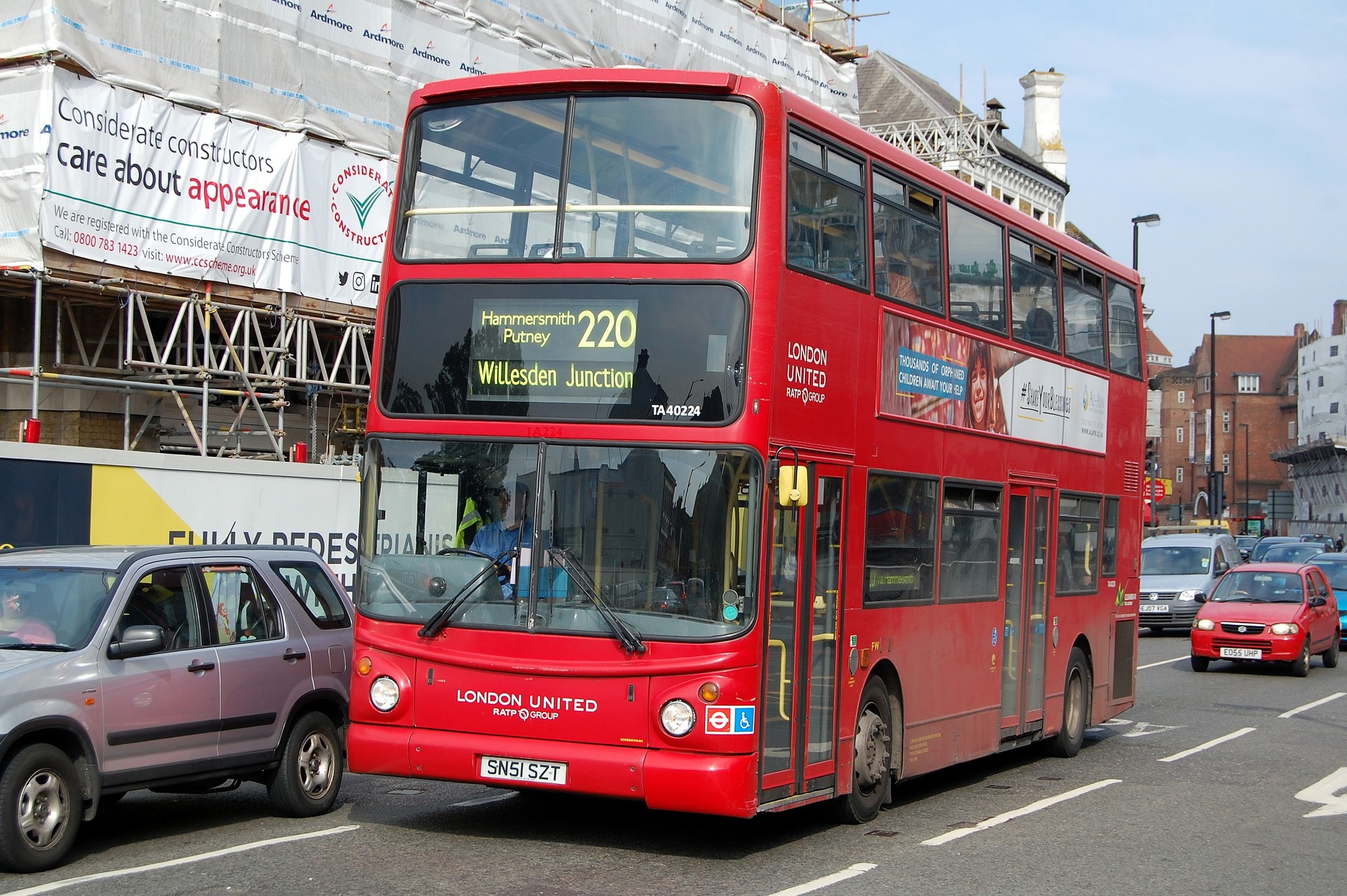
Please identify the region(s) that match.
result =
[1189,519,1232,535]
[1138,524,1245,635]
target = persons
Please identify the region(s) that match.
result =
[466,483,568,605]
[960,338,1005,434]
[1238,529,1345,552]
[0,580,57,645]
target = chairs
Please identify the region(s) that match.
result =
[687,239,742,259]
[235,582,276,643]
[1284,576,1302,601]
[786,225,1055,349]
[467,243,520,258]
[528,242,585,258]
[85,586,179,657]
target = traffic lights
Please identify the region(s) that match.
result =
[1145,439,1154,473]
[1217,490,1226,511]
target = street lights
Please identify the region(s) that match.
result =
[1132,213,1161,380]
[1206,311,1232,519]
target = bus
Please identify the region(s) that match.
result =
[346,68,1145,822]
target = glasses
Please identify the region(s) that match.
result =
[5,591,30,599]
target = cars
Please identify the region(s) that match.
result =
[1236,535,1339,565]
[1303,552,1347,652]
[1190,559,1341,677]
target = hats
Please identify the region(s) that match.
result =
[492,482,530,497]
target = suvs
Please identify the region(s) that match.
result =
[0,544,355,873]
